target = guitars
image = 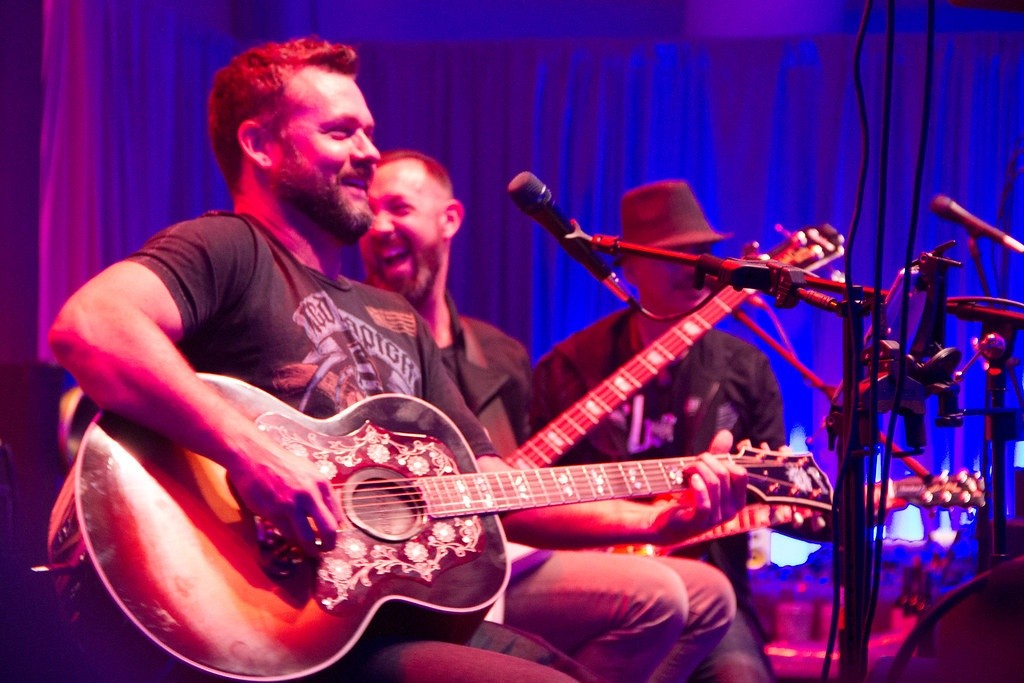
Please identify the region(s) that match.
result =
[572,465,986,557]
[501,223,845,471]
[47,372,835,683]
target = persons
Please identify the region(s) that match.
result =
[48,35,749,683]
[355,150,734,683]
[532,181,786,683]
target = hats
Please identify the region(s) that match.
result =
[610,181,733,266]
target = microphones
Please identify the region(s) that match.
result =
[930,194,1024,255]
[507,171,639,311]
